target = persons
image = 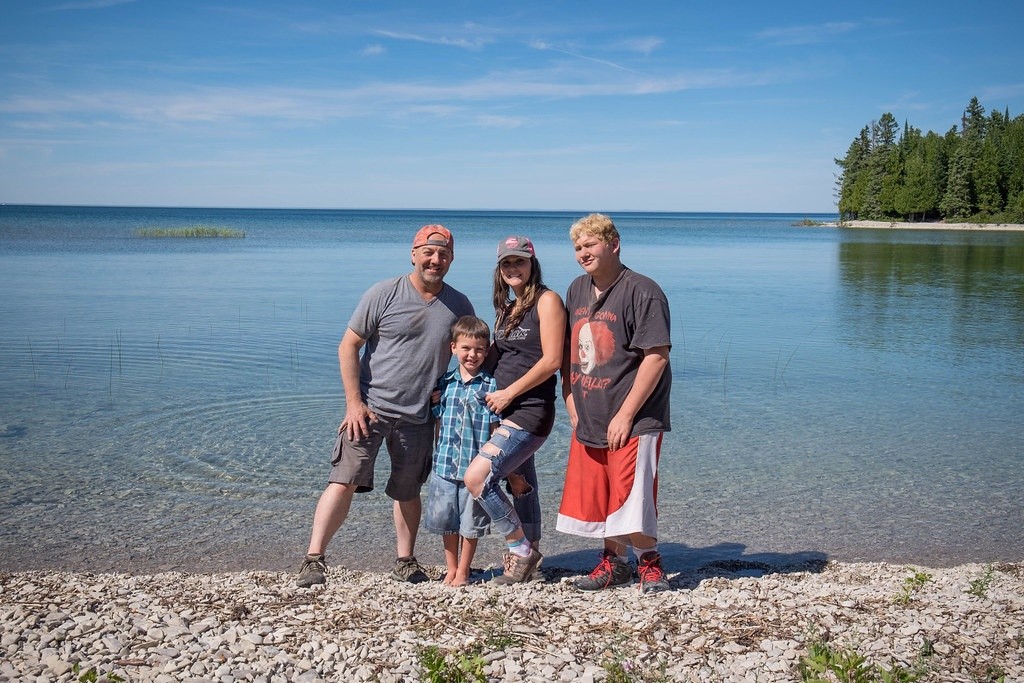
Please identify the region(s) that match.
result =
[430,234,569,586]
[296,222,478,589]
[560,211,674,594]
[426,314,503,589]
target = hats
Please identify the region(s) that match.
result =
[412,224,454,266]
[496,235,535,265]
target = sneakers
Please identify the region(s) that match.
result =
[391,556,429,584]
[491,543,545,586]
[638,551,670,595]
[573,547,636,593]
[296,553,329,588]
